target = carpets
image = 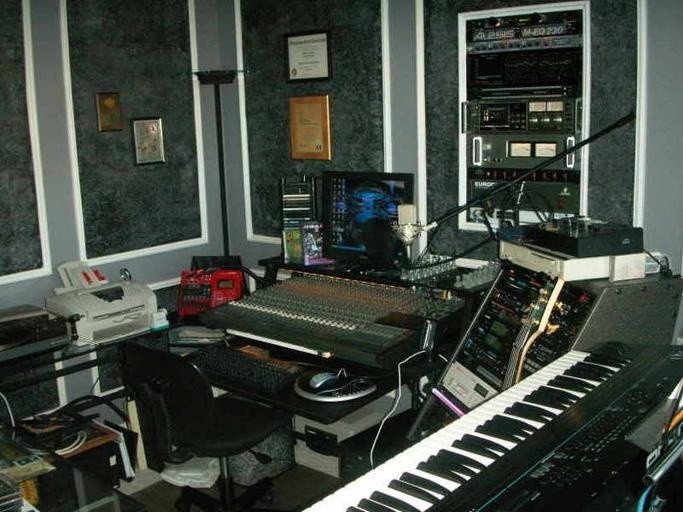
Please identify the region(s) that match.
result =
[121,462,340,512]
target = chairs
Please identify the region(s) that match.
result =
[121,342,278,512]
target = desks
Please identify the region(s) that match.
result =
[182,257,494,424]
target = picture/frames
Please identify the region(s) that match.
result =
[94,92,123,132]
[130,117,166,166]
[288,94,331,161]
[284,29,333,83]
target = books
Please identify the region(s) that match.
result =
[280,226,306,266]
[300,223,337,266]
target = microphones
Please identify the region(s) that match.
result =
[394,200,418,262]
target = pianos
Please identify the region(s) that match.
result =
[302,342,683,511]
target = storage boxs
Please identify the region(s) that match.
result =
[218,392,296,488]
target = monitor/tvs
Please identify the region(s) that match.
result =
[322,171,414,260]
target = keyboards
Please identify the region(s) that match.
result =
[183,344,297,396]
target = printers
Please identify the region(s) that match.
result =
[44,260,158,341]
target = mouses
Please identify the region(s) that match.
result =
[309,371,338,389]
